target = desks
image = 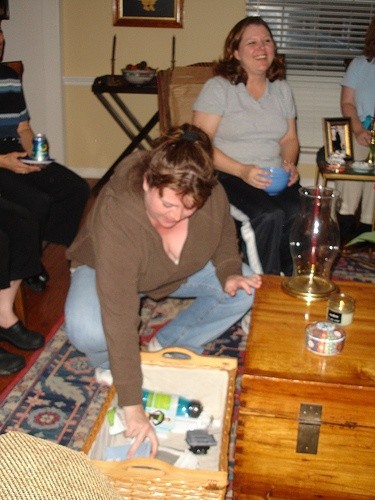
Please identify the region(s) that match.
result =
[315,146,375,233]
[232,275,375,500]
[92,76,160,194]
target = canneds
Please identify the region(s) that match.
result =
[31,133,51,160]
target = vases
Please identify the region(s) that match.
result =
[286,188,341,302]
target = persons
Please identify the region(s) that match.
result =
[65,120,263,457]
[197,17,314,276]
[0,28,90,375]
[329,16,375,251]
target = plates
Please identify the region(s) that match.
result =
[350,163,373,174]
[20,159,52,166]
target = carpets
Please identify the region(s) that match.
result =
[0,249,375,500]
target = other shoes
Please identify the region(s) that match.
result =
[95,368,113,387]
[0,319,44,349]
[148,336,171,358]
[0,347,25,375]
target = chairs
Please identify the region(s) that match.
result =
[158,67,215,134]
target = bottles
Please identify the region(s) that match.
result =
[280,185,341,302]
[327,293,355,326]
[365,136,375,175]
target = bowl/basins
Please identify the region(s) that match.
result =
[259,167,289,196]
[121,68,155,84]
[304,322,347,356]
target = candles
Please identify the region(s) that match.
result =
[173,36,175,61]
[112,36,116,58]
[331,301,353,325]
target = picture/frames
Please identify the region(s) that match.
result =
[112,0,184,28]
[323,117,353,161]
